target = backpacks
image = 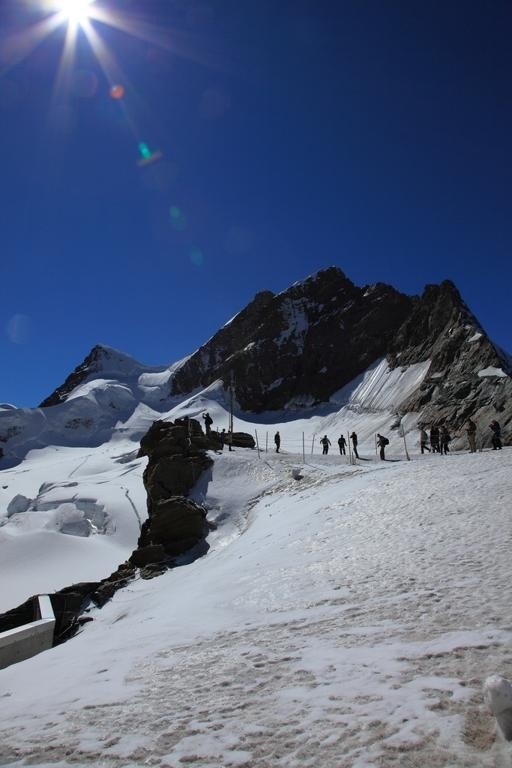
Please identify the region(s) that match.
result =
[381,436,389,445]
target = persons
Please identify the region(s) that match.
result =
[434,428,440,452]
[226,431,235,451]
[429,425,438,452]
[439,426,450,455]
[320,434,332,454]
[465,417,477,454]
[274,431,281,453]
[489,419,503,451]
[377,433,385,460]
[203,412,213,435]
[221,428,225,449]
[350,431,359,458]
[419,427,430,454]
[338,435,347,455]
